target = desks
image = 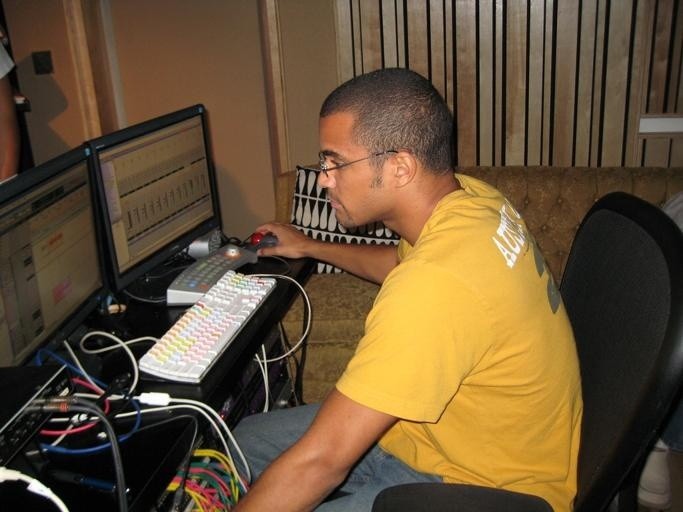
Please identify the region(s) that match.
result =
[0,239,318,512]
[371,191,683,511]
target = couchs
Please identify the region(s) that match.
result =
[275,165,682,403]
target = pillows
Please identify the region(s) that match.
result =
[291,165,401,273]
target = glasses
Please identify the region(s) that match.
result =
[317,150,400,179]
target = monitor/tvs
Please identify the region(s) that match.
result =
[0,142,105,366]
[88,103,223,288]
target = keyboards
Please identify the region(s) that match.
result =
[134,267,277,386]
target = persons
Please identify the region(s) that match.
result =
[228,68,584,512]
[636,193,683,511]
[1,38,20,183]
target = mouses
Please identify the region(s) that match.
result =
[244,227,281,250]
